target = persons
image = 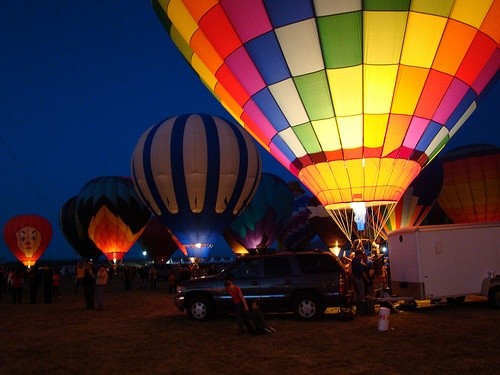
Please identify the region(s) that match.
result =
[224,280,249,335]
[95,265,108,311]
[4,259,59,304]
[125,264,158,291]
[73,262,86,294]
[168,264,193,292]
[352,249,390,316]
[252,301,277,334]
[83,262,97,309]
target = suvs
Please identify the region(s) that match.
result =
[172,247,347,321]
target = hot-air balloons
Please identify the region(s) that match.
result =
[432,144,500,223]
[151,0,500,310]
[129,112,442,277]
[58,175,154,293]
[3,213,53,278]
